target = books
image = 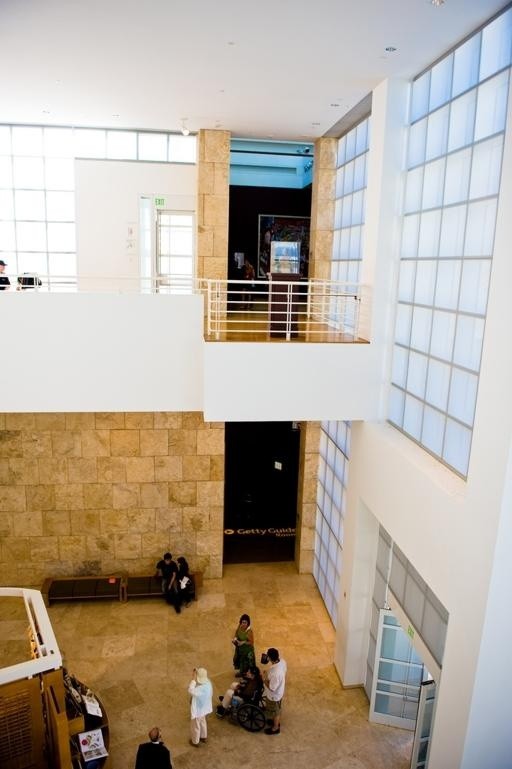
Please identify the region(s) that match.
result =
[77,728,109,762]
[80,694,103,717]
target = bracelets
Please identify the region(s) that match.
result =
[263,678,267,682]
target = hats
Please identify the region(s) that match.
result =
[196,667,208,684]
[0,261,7,266]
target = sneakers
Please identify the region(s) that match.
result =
[167,600,192,613]
[199,738,207,743]
[188,739,200,748]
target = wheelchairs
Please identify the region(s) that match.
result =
[216,684,267,734]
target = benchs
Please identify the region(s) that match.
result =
[121,572,205,604]
[38,573,124,607]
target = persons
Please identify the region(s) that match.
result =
[1,260,11,292]
[216,666,262,717]
[231,613,254,679]
[133,726,174,769]
[174,556,193,614]
[187,667,214,748]
[259,645,287,736]
[156,553,182,605]
[239,260,256,309]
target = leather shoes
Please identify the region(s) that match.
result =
[266,717,280,726]
[264,727,280,735]
